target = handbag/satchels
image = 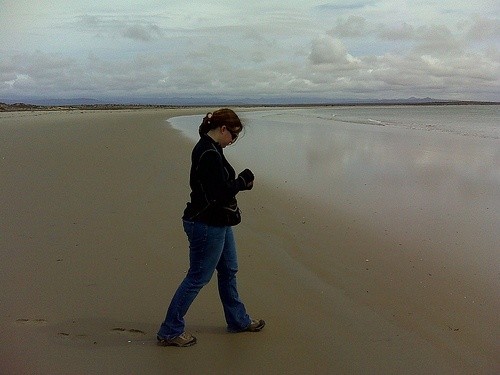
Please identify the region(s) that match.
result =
[209,195,241,227]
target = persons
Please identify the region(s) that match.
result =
[156,108,264,346]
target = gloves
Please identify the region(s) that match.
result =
[240,168,254,184]
[242,183,253,190]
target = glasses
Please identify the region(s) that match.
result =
[227,127,238,142]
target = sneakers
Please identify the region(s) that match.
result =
[248,319,266,332]
[157,331,196,347]
